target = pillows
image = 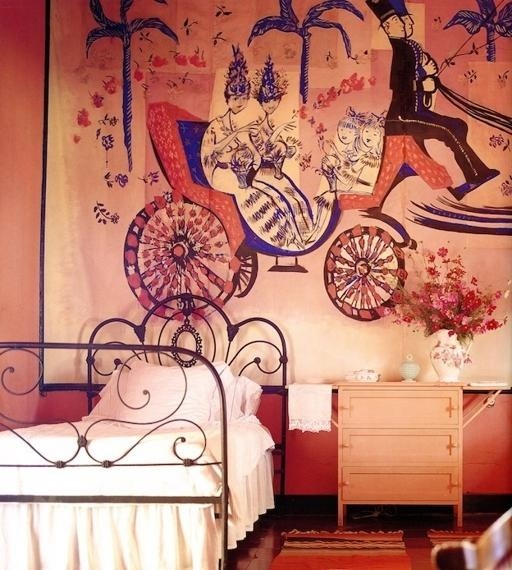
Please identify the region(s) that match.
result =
[91,360,260,425]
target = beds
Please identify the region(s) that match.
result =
[0,293,290,569]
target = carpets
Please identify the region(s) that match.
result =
[274,526,484,570]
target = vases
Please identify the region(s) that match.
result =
[432,326,464,383]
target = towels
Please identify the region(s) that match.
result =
[288,381,332,433]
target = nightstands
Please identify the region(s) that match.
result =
[327,379,467,528]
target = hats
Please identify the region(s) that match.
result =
[388,0,413,17]
[364,0,398,31]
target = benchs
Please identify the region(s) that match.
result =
[147,100,247,253]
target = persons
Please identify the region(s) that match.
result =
[201,0,500,249]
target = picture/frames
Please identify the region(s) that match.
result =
[38,0,512,395]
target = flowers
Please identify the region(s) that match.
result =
[387,242,511,345]
